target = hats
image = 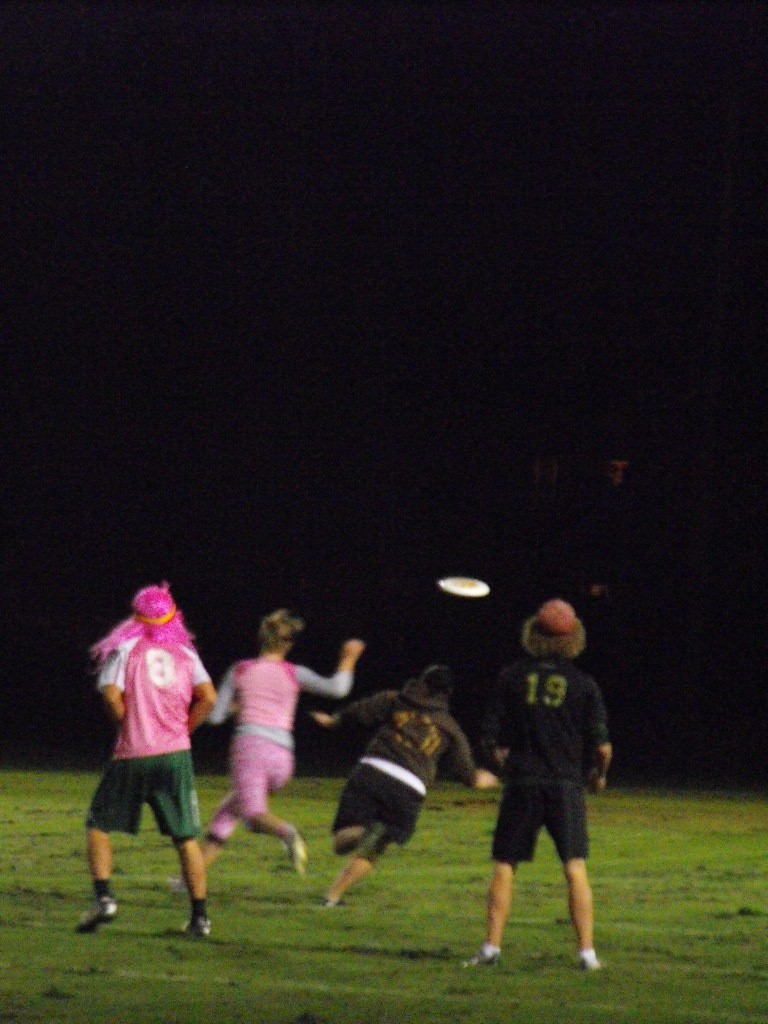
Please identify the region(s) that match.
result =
[259,609,304,640]
[522,598,586,657]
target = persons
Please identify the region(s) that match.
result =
[167,610,366,893]
[312,664,498,908]
[463,597,611,972]
[77,586,217,936]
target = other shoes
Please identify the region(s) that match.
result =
[289,836,309,874]
[469,950,497,966]
[357,823,386,857]
[73,898,117,934]
[581,959,602,971]
[181,915,210,937]
[323,899,344,907]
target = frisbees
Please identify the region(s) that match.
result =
[436,576,490,598]
